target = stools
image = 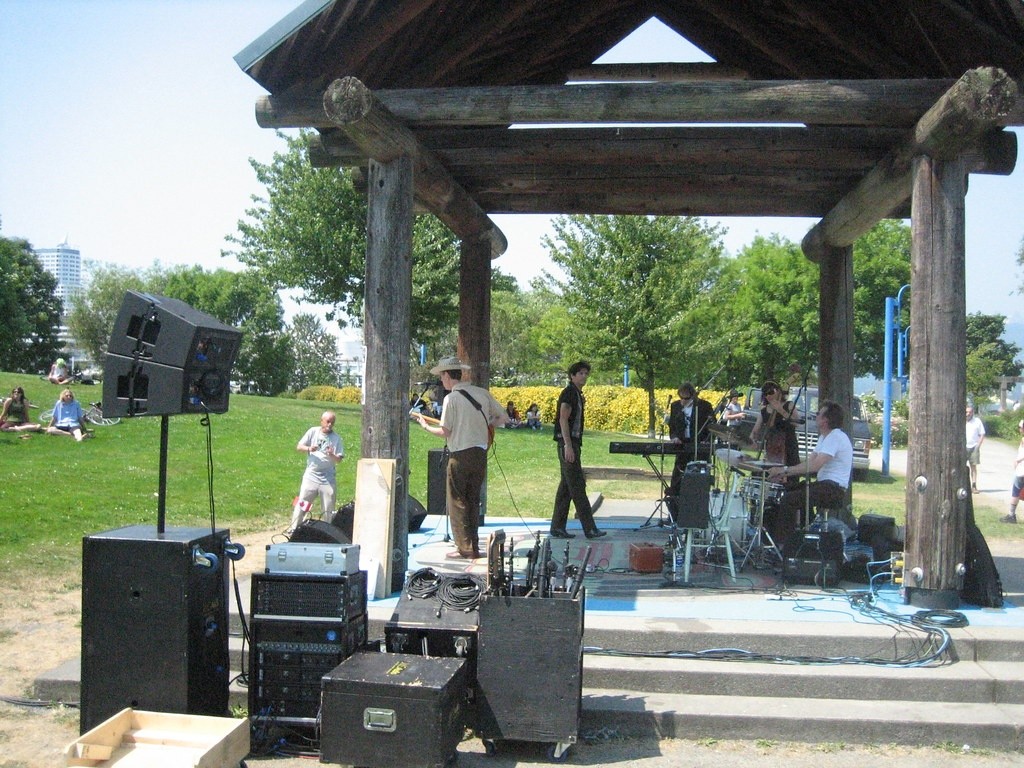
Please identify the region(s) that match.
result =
[814,501,842,533]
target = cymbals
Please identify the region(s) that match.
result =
[706,420,755,451]
[743,461,784,467]
[714,442,755,470]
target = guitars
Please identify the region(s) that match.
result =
[410,412,495,449]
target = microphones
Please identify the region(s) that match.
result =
[414,380,443,385]
[667,395,672,408]
[726,393,744,399]
[783,418,806,425]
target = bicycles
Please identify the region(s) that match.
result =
[39,401,122,426]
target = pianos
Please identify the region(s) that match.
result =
[607,436,739,532]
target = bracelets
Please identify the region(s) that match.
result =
[308,447,309,452]
[424,424,428,430]
[564,443,572,448]
[784,466,788,475]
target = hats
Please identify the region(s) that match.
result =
[727,390,738,397]
[430,356,471,374]
[57,358,65,369]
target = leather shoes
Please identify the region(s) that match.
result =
[550,530,575,538]
[446,551,479,559]
[586,528,608,539]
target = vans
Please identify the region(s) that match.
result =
[739,384,871,480]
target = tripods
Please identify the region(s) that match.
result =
[706,439,785,574]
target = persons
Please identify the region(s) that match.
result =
[288,410,344,537]
[0,387,41,431]
[717,390,745,444]
[525,404,544,431]
[999,419,1024,523]
[749,380,801,527]
[47,388,89,442]
[966,407,985,494]
[48,358,74,384]
[764,402,852,543]
[410,393,442,420]
[667,383,717,522]
[417,357,509,560]
[505,401,522,429]
[550,362,608,538]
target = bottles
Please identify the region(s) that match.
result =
[676,550,684,579]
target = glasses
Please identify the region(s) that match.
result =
[13,391,21,393]
[816,412,822,416]
[764,391,774,396]
[680,397,690,401]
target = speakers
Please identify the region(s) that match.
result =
[408,494,427,533]
[427,448,487,514]
[101,292,242,419]
[80,522,230,738]
[288,504,355,546]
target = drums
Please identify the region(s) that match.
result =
[739,478,785,503]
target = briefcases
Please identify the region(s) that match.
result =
[629,541,664,572]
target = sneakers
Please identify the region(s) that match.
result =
[1000,514,1016,523]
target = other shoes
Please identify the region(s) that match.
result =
[972,488,979,494]
